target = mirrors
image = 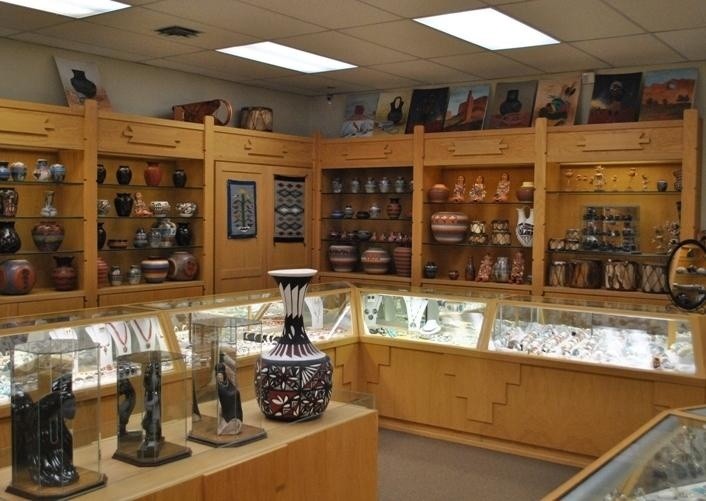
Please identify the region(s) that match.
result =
[665,239,706,310]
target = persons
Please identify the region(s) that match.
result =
[469,176,487,203]
[492,172,512,203]
[128,191,154,218]
[447,176,466,202]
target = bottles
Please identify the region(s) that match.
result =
[39,189,59,218]
[343,203,353,219]
[331,176,405,194]
[127,265,141,286]
[368,197,402,218]
[465,254,475,281]
[108,264,123,286]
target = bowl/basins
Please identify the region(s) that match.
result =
[448,270,459,280]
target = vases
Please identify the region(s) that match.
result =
[0,98,212,319]
[253,266,334,422]
[316,109,703,294]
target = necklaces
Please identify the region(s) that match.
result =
[408,301,423,329]
[364,296,380,321]
[422,325,438,333]
[0,297,346,399]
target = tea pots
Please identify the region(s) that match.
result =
[515,204,533,248]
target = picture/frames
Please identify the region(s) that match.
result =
[228,179,260,239]
[273,177,305,243]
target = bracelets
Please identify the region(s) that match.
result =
[368,326,397,339]
[496,322,677,371]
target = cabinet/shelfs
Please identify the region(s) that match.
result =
[212,115,316,296]
[0,281,706,469]
[0,395,380,501]
[672,246,705,301]
[537,406,706,501]
